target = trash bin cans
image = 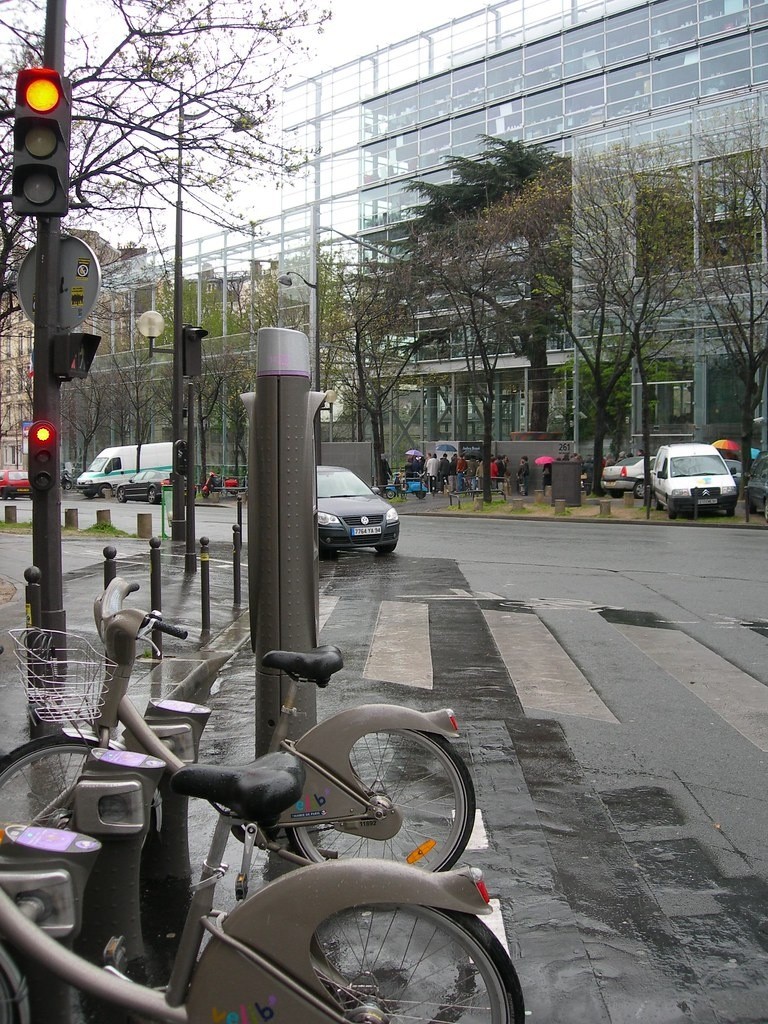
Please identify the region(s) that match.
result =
[551,461,581,507]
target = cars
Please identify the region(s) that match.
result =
[116,470,197,505]
[724,460,741,487]
[744,454,768,523]
[600,455,655,499]
[750,451,768,475]
[0,470,33,500]
[316,464,400,561]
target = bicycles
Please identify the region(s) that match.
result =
[0,751,525,1024]
[0,577,476,902]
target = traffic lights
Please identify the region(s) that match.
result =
[53,333,102,379]
[174,439,189,476]
[183,325,208,377]
[27,421,55,490]
[12,68,72,217]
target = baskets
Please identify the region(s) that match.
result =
[8,628,118,721]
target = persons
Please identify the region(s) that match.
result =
[60,467,71,486]
[568,449,644,490]
[516,456,529,496]
[731,467,737,474]
[721,452,739,460]
[224,477,238,495]
[380,449,512,495]
[541,463,553,495]
[206,468,222,494]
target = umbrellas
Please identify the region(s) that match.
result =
[711,439,742,451]
[535,455,555,465]
[751,448,763,458]
[405,449,425,457]
[436,444,457,454]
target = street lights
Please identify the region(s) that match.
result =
[278,271,321,466]
[325,390,337,442]
[137,310,186,541]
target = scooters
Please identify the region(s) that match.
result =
[386,471,429,500]
[202,472,239,498]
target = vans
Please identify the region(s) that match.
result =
[652,442,738,520]
[76,441,173,498]
